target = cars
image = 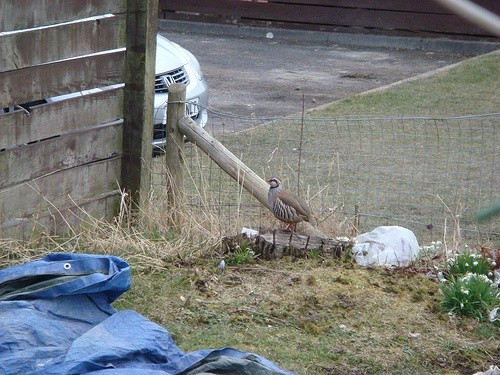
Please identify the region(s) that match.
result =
[1,33,210,154]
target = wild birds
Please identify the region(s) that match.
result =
[265,177,317,234]
